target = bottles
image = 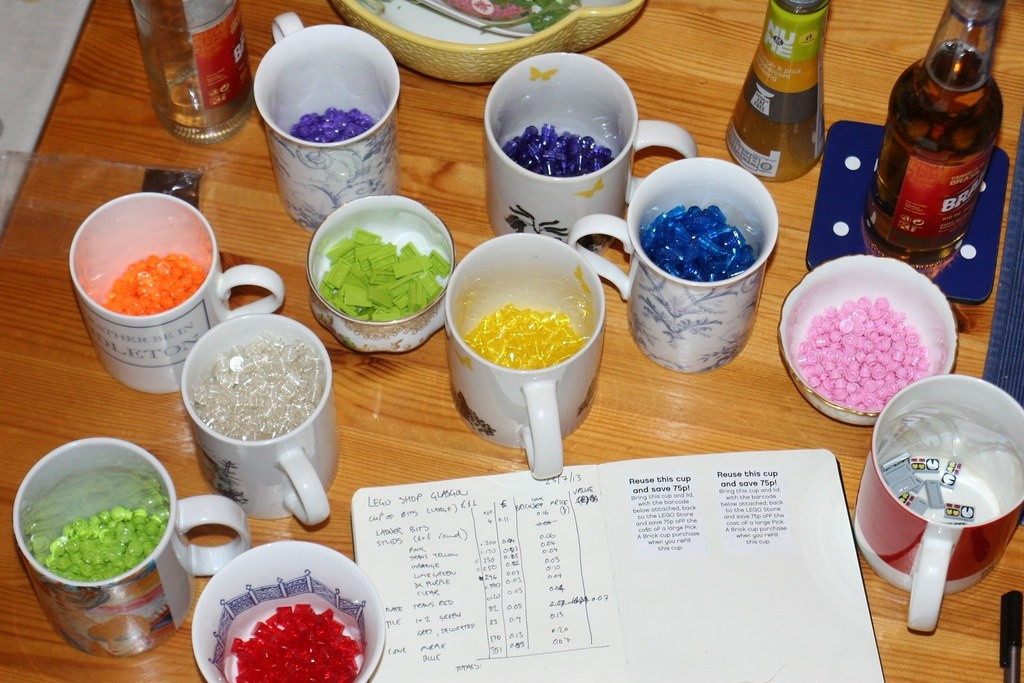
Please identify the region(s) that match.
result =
[863,0,1005,264]
[131,0,252,144]
[725,0,832,182]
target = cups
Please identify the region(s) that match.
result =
[12,438,251,655]
[445,232,607,482]
[67,193,285,394]
[852,375,1024,634]
[190,541,387,683]
[253,12,401,227]
[569,158,777,372]
[484,52,696,250]
[181,313,339,524]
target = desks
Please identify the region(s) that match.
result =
[0,1,1024,683]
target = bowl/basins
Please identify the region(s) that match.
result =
[778,254,959,425]
[305,194,454,354]
[330,0,646,84]
